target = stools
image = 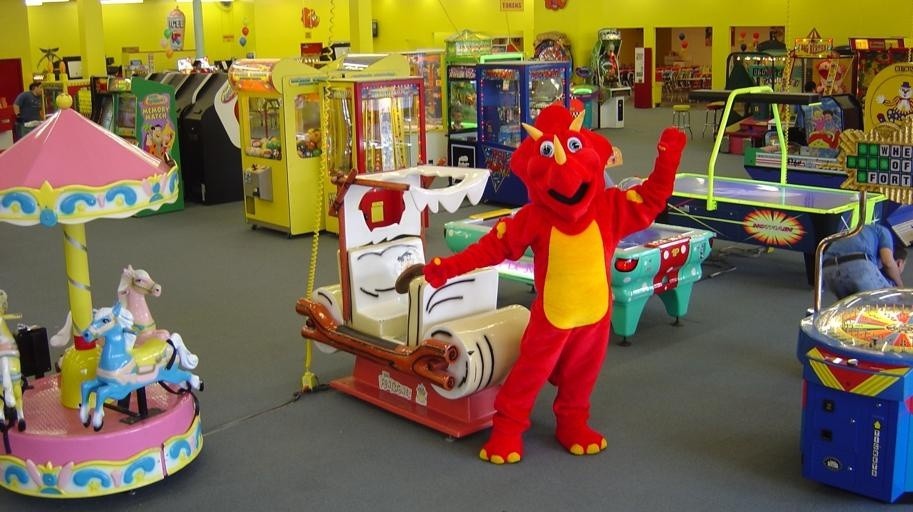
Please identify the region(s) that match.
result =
[702,100,726,137]
[672,104,693,139]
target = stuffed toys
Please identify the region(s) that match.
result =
[423,104,687,465]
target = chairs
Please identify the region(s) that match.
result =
[619,66,713,104]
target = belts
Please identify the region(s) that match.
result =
[822,254,865,268]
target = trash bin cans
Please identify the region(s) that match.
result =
[655,73,664,107]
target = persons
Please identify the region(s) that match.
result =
[820,223,906,301]
[13,82,45,124]
